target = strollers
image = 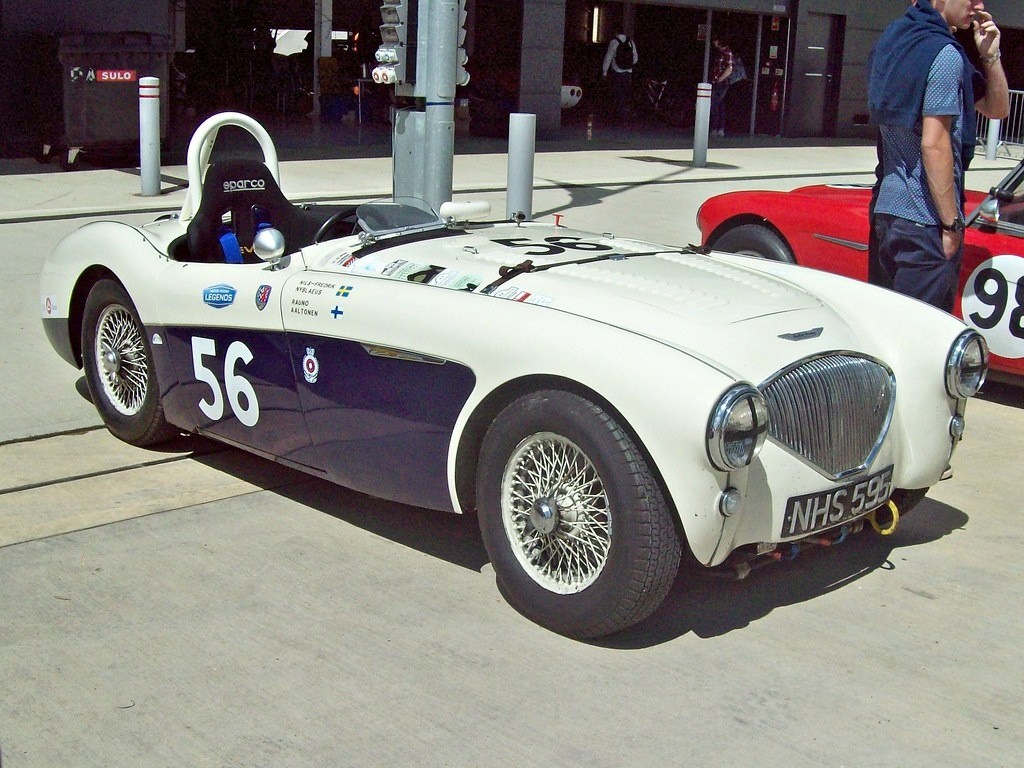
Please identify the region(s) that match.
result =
[632,69,680,124]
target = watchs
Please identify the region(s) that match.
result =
[941,217,963,233]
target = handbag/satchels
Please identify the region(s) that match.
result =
[722,49,747,84]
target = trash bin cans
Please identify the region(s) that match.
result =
[57,31,177,150]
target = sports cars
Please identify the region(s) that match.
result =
[32,111,993,643]
[693,151,1024,394]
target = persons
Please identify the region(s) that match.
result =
[602,26,638,123]
[709,32,734,137]
[867,0,1010,312]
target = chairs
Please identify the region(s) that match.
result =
[188,159,336,264]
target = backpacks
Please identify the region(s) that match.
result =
[614,36,633,69]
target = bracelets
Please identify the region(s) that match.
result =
[979,49,1001,63]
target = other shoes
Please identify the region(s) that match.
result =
[711,129,724,137]
